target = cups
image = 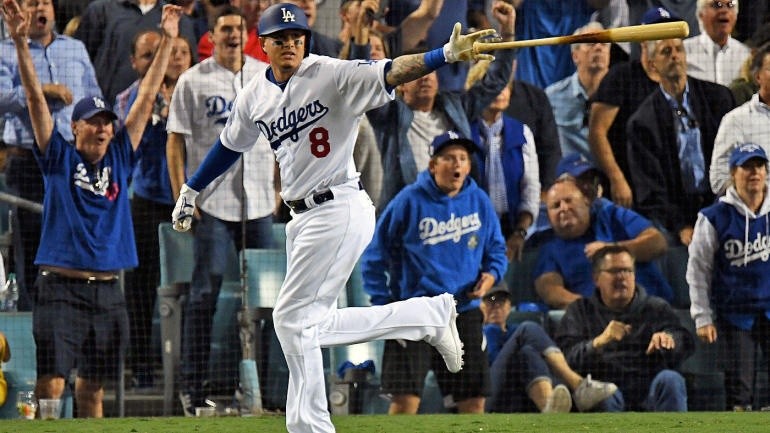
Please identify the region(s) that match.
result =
[38,399,61,421]
[17,391,37,419]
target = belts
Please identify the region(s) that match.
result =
[284,180,364,214]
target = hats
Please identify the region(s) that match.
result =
[481,281,512,301]
[71,97,120,123]
[555,153,603,182]
[428,132,482,160]
[257,2,312,38]
[728,142,770,168]
[639,6,684,25]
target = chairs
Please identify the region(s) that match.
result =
[0,311,75,418]
[158,222,435,417]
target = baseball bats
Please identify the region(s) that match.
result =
[474,21,690,55]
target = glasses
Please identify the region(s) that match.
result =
[707,2,736,9]
[672,104,697,134]
[597,265,635,275]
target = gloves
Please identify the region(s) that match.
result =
[170,183,200,232]
[442,22,499,64]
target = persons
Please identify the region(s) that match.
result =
[0,1,770,433]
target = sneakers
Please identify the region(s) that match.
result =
[542,384,573,414]
[572,374,617,410]
[435,291,465,375]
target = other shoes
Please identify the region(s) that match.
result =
[178,390,201,418]
